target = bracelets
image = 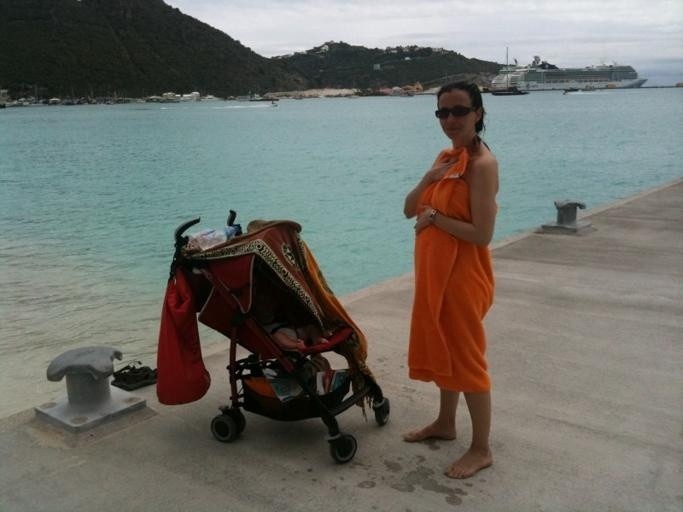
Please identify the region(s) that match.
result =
[429,210,439,222]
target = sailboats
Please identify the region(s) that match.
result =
[492,46,530,96]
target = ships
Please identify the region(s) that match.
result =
[492,59,648,90]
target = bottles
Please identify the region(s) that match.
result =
[199,226,241,251]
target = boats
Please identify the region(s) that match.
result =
[390,86,402,97]
[407,90,416,97]
[0,86,280,107]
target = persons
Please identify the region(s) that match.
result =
[251,296,335,349]
[403,79,507,479]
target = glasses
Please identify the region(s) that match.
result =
[435,106,475,118]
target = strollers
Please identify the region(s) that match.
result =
[174,209,390,464]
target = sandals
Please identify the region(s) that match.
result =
[111,365,157,390]
